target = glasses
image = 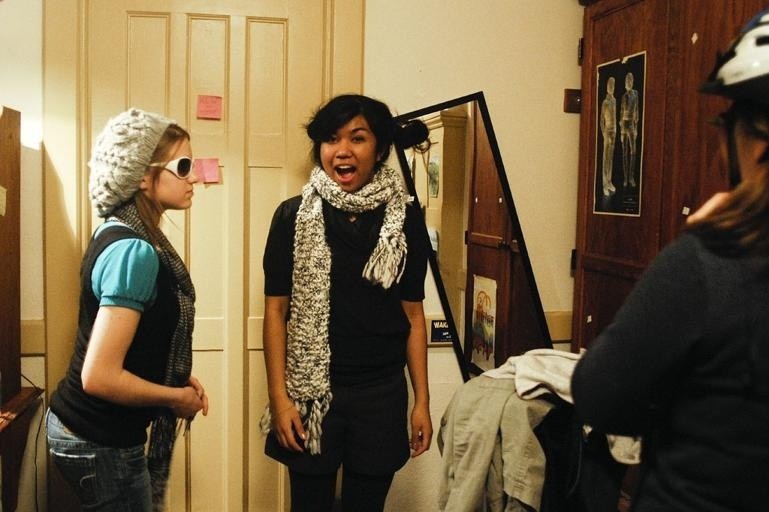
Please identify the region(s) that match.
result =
[148,156,191,180]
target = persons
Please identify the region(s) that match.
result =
[260,91,435,511]
[41,104,210,511]
[616,71,641,189]
[568,9,767,512]
[599,76,619,198]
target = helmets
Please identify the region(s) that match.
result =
[699,6,769,101]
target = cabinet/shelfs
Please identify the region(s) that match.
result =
[462,102,552,381]
[577,2,768,385]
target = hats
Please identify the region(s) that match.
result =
[86,108,178,219]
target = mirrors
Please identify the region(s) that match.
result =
[389,89,554,388]
